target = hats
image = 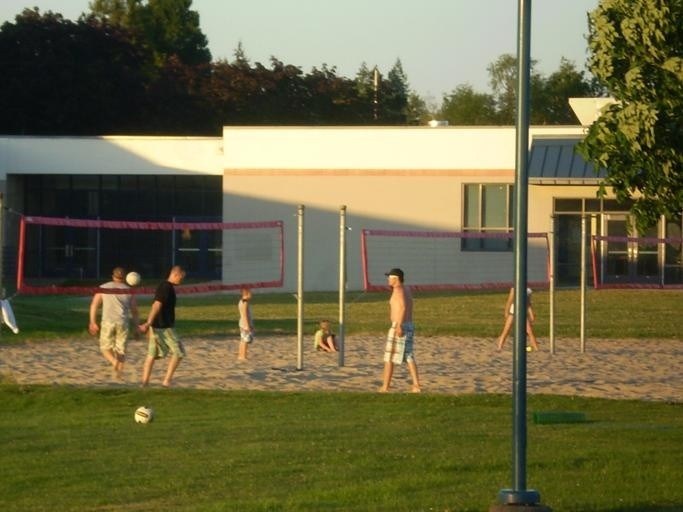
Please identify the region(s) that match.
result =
[384,268,404,283]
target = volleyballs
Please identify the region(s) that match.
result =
[135,406,153,424]
[126,272,142,287]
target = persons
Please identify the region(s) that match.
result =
[1,286,19,336]
[136,264,190,389]
[498,285,540,354]
[378,268,425,393]
[311,318,342,354]
[87,265,142,379]
[235,285,255,363]
